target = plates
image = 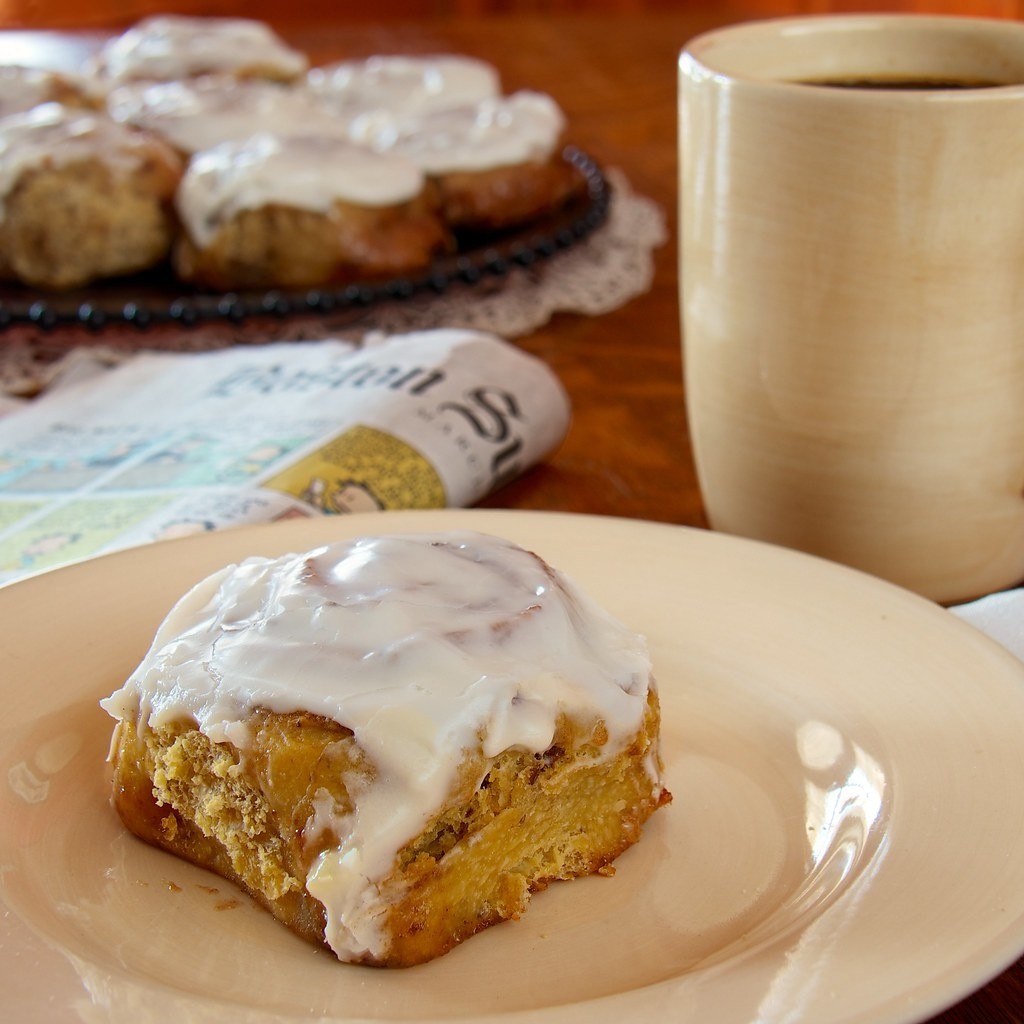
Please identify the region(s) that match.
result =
[0,508,1024,1024]
[0,137,610,324]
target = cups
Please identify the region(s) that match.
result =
[676,14,1024,613]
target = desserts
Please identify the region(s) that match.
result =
[105,531,666,951]
[0,20,606,285]
